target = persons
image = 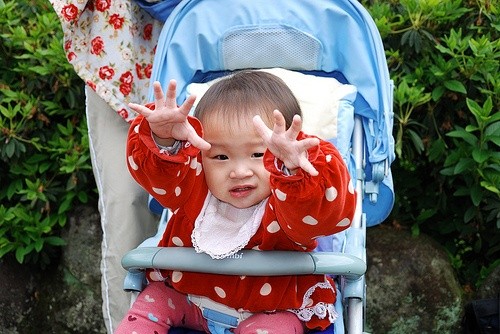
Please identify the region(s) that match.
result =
[113,70,357,334]
[48,0,164,334]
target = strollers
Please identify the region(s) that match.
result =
[121,1,396,334]
